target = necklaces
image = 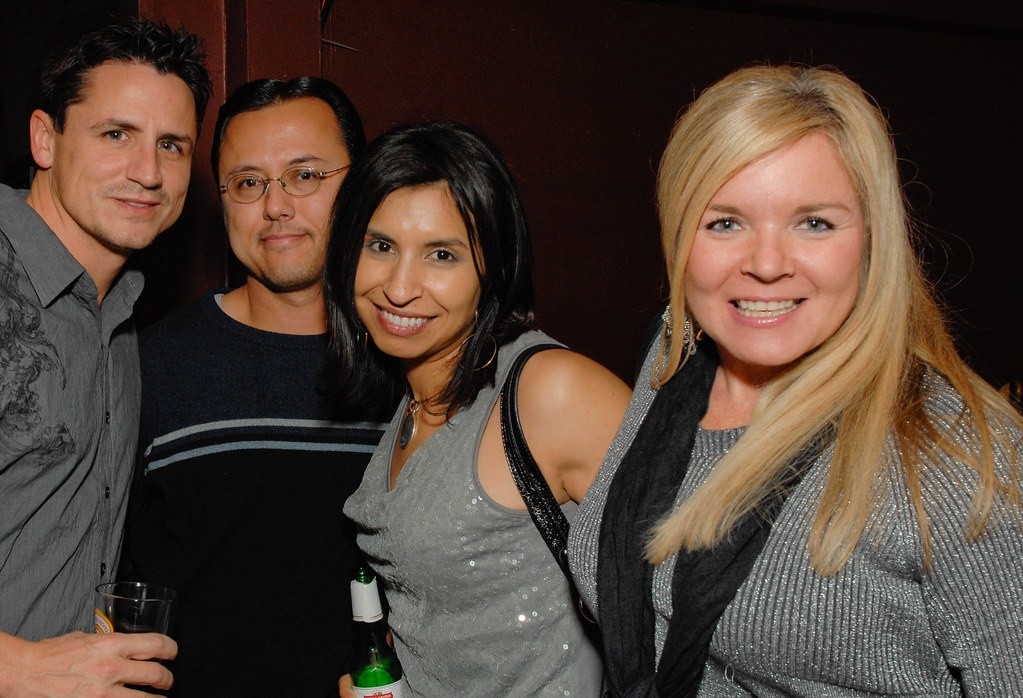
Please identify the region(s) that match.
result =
[400,389,446,449]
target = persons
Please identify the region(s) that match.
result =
[0,14,213,698]
[565,63,1023,698]
[321,120,634,698]
[111,76,408,698]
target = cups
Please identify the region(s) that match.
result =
[94,582,179,694]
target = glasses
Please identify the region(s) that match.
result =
[220,164,352,204]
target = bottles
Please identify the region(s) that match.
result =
[348,563,403,698]
